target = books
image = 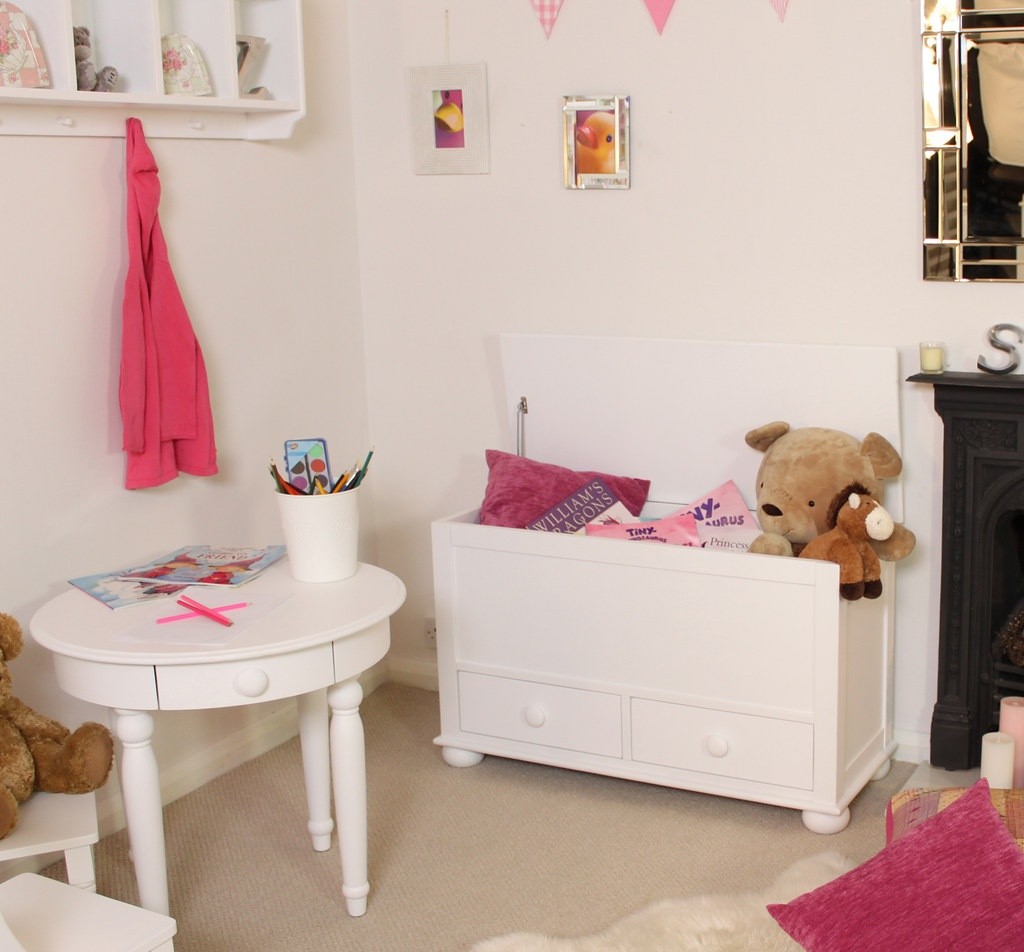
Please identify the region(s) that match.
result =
[66,544,287,613]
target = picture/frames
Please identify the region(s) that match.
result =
[413,65,491,176]
[563,95,631,190]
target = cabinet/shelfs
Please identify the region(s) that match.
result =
[0,0,307,141]
[427,331,907,835]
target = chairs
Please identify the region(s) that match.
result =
[0,790,98,894]
[886,787,1024,850]
[0,873,177,952]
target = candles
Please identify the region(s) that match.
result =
[980,697,1023,789]
[921,344,943,369]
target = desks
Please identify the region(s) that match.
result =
[30,553,406,919]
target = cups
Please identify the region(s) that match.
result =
[274,476,362,583]
[919,340,944,375]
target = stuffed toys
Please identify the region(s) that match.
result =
[0,612,114,842]
[744,422,902,559]
[798,481,895,601]
[73,26,118,92]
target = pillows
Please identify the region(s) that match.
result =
[768,778,1024,952]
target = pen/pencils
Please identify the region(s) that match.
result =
[268,458,308,495]
[309,472,318,495]
[157,602,252,624]
[181,595,233,624]
[315,478,325,494]
[354,445,375,488]
[330,463,368,493]
[178,600,231,626]
